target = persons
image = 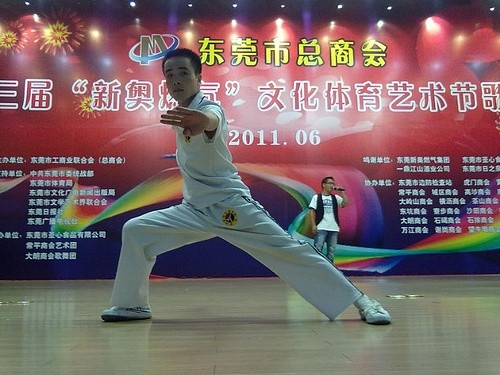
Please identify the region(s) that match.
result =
[308,176,349,263]
[101,48,392,325]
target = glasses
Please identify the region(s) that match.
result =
[324,182,335,186]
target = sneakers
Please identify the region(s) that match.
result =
[359,299,391,324]
[101,305,152,322]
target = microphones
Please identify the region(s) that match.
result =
[331,186,345,191]
